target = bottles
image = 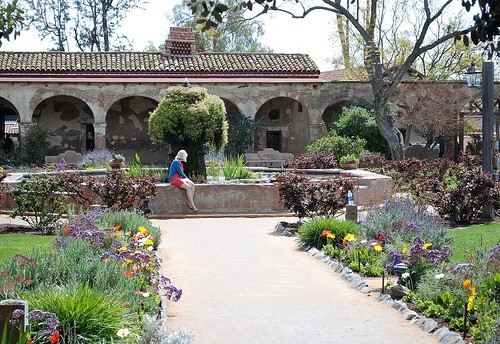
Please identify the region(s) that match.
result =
[348,190,354,205]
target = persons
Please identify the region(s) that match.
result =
[3,134,14,155]
[168,150,198,212]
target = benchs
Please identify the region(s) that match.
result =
[245,149,294,167]
[45,151,83,166]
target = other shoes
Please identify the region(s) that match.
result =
[187,204,193,210]
[193,209,198,212]
